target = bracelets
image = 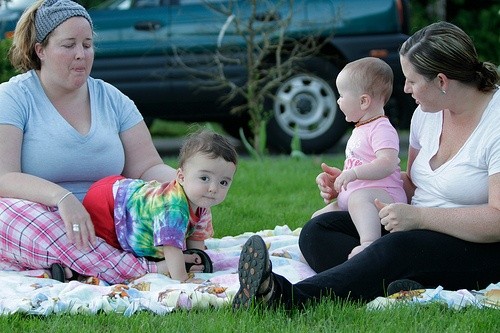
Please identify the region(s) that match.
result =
[57,192,73,206]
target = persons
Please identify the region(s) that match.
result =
[0,0,213,285]
[82,130,239,284]
[231,21,500,316]
[310,57,407,260]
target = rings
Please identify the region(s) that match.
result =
[72,224,80,232]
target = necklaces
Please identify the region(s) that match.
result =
[355,116,387,127]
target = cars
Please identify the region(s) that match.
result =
[0,1,416,154]
